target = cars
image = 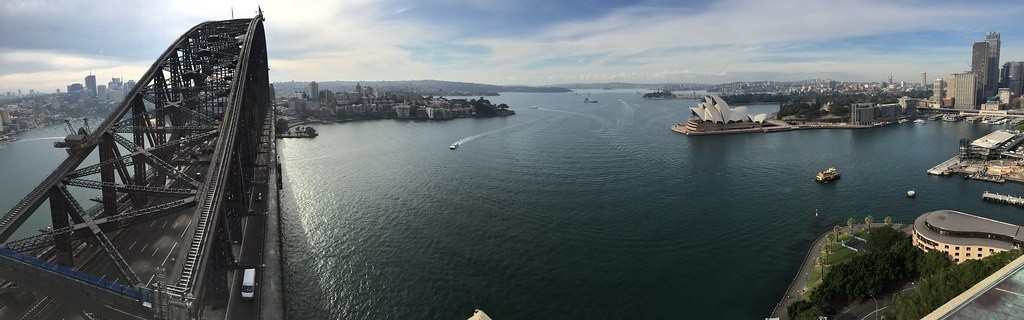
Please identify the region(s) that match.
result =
[256,192,262,201]
[196,172,201,178]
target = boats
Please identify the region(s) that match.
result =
[450,143,459,149]
[816,167,841,185]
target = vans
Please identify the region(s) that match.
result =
[241,268,256,300]
